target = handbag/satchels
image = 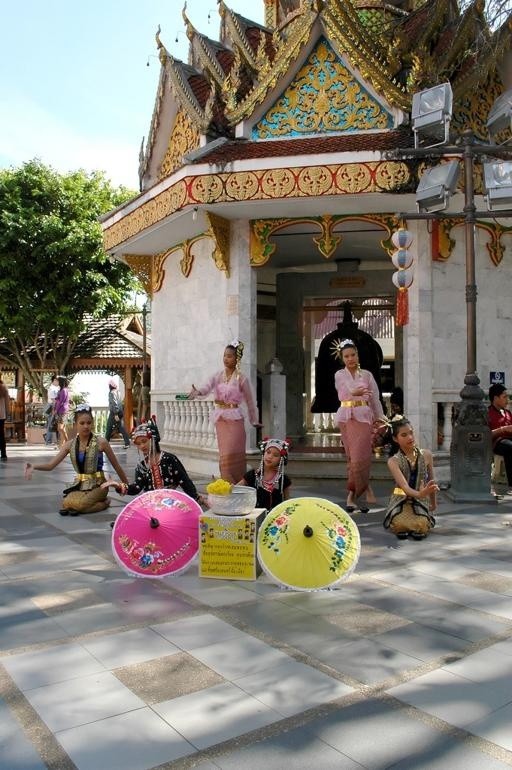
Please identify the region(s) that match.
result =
[112,411,123,421]
[48,424,58,432]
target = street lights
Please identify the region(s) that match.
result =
[411,81,512,506]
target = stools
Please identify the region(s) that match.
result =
[493,454,508,486]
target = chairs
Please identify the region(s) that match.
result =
[4,399,24,443]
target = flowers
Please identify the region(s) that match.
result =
[280,449,287,456]
[119,534,192,573]
[147,430,152,440]
[151,415,157,422]
[392,413,403,422]
[139,417,146,424]
[76,403,90,411]
[285,439,292,445]
[317,504,353,571]
[264,437,268,441]
[231,338,239,348]
[339,338,353,348]
[124,488,193,521]
[263,504,301,556]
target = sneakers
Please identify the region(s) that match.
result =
[123,445,130,449]
[45,442,55,446]
[0,456,7,460]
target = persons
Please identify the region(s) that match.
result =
[383,415,439,541]
[0,372,11,460]
[192,340,265,484]
[486,385,512,495]
[48,376,60,445]
[52,377,70,447]
[378,389,404,454]
[105,380,131,448]
[331,338,386,514]
[233,438,291,513]
[24,403,129,516]
[99,421,212,527]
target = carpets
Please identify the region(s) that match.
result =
[288,446,345,454]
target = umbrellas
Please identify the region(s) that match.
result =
[258,496,361,589]
[112,488,204,578]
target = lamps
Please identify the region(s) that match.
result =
[192,207,198,220]
[109,252,115,264]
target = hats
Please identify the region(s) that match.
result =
[257,437,290,465]
[131,415,160,444]
[110,381,117,388]
